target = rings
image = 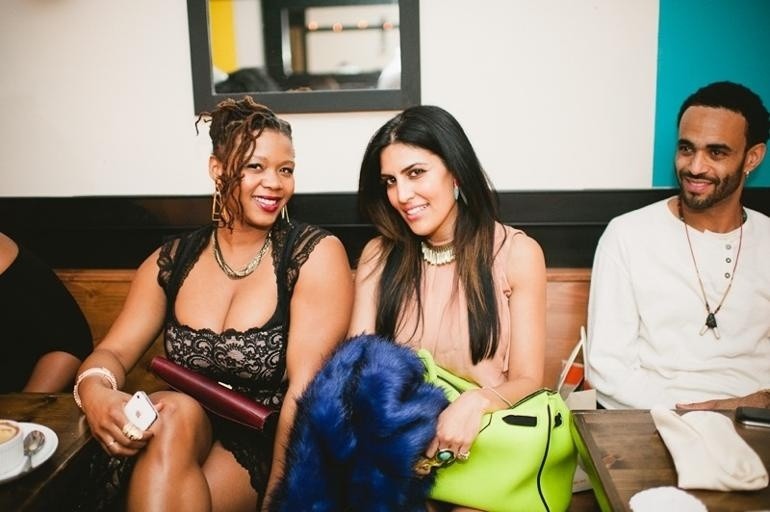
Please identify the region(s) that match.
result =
[122,423,143,443]
[457,448,471,463]
[105,440,117,449]
[437,447,455,465]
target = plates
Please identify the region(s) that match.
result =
[0,419,59,484]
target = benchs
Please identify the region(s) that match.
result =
[50,263,590,414]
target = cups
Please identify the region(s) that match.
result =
[0,419,24,468]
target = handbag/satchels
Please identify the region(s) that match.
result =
[148,354,280,435]
[415,349,577,512]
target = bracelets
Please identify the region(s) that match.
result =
[71,367,118,409]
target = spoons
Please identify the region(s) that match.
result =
[20,430,46,474]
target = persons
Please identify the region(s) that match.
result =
[344,105,549,512]
[72,97,355,511]
[579,80,769,418]
[0,232,94,395]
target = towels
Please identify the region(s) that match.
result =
[646,400,768,497]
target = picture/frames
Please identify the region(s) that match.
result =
[185,0,423,119]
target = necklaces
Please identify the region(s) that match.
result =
[421,236,458,267]
[211,226,272,281]
[678,201,743,340]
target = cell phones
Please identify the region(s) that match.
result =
[734,406,770,428]
[123,390,158,432]
